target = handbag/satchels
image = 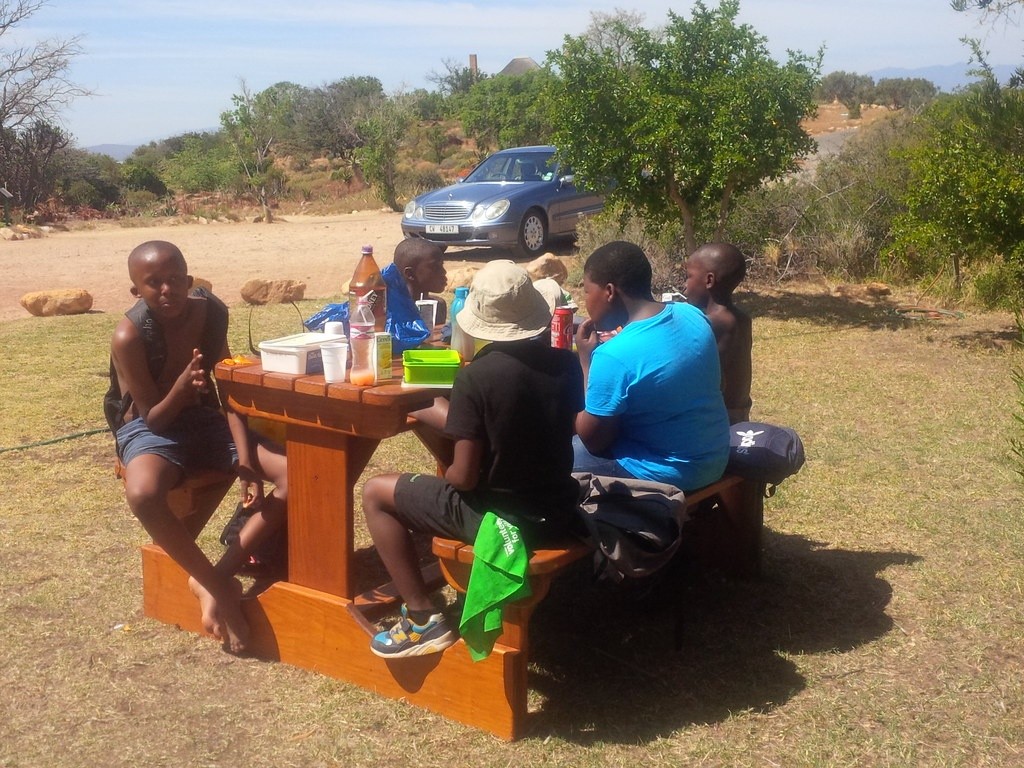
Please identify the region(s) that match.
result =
[728,422,806,499]
[564,472,687,583]
[303,262,429,353]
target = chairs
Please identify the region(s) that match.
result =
[519,162,543,181]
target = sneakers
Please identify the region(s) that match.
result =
[400,603,409,617]
[369,612,456,659]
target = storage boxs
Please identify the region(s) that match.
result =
[255,332,346,375]
[402,348,462,385]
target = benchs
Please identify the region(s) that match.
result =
[114,416,421,645]
[431,467,768,744]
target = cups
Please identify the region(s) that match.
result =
[324,322,344,334]
[320,343,348,383]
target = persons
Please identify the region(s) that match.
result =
[299,237,585,659]
[571,241,730,493]
[683,241,753,428]
[110,240,288,653]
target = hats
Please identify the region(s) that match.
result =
[456,258,555,342]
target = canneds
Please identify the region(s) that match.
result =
[551,308,572,353]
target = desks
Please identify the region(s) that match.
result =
[214,322,450,713]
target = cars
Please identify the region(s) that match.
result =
[401,145,649,258]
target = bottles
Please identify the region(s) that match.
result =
[349,245,386,332]
[450,287,475,361]
[349,297,377,386]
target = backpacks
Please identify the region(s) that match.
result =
[103,285,228,439]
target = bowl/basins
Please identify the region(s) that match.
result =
[403,350,461,385]
[258,332,348,375]
